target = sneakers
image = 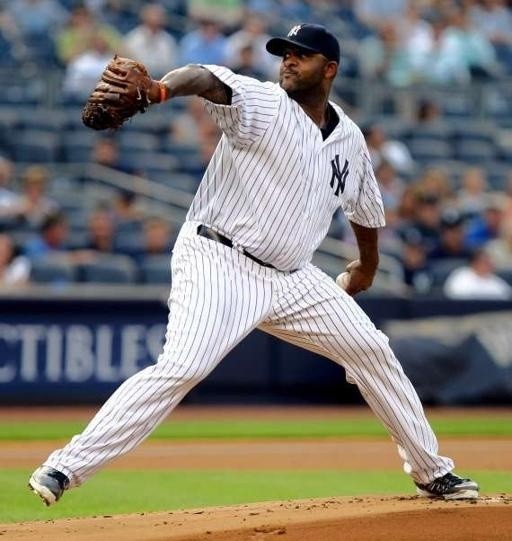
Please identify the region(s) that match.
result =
[27,465,69,507]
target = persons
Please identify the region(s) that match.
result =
[1,158,178,293]
[0,0,512,173]
[28,24,480,509]
[338,159,512,299]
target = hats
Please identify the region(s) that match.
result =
[266,23,340,65]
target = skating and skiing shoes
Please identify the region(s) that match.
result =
[413,472,479,500]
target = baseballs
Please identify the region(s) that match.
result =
[335,271,351,291]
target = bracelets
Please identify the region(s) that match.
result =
[154,79,171,103]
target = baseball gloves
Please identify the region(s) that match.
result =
[81,55,154,130]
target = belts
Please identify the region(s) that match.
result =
[197,224,275,268]
